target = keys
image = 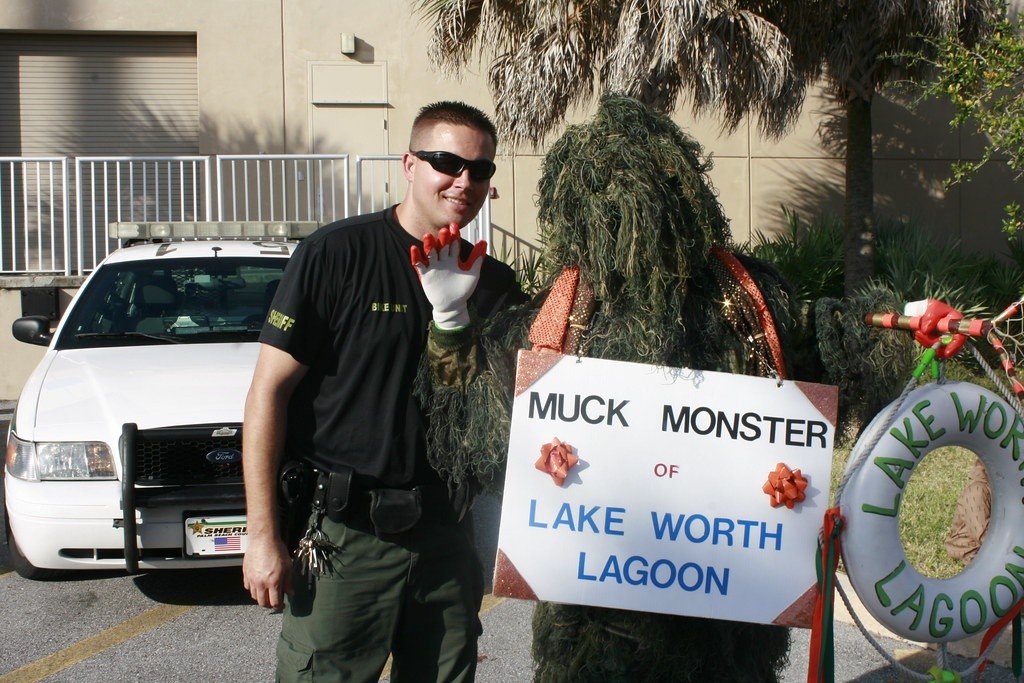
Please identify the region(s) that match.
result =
[291,536,341,580]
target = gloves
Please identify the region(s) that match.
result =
[904,298,967,358]
[410,221,487,330]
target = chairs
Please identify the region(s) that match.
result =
[124,274,281,336]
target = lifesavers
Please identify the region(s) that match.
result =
[839,383,1024,639]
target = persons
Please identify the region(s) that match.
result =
[412,97,836,683]
[241,101,530,683]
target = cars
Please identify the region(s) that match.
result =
[2,240,297,581]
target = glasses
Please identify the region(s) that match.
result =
[410,149,497,181]
